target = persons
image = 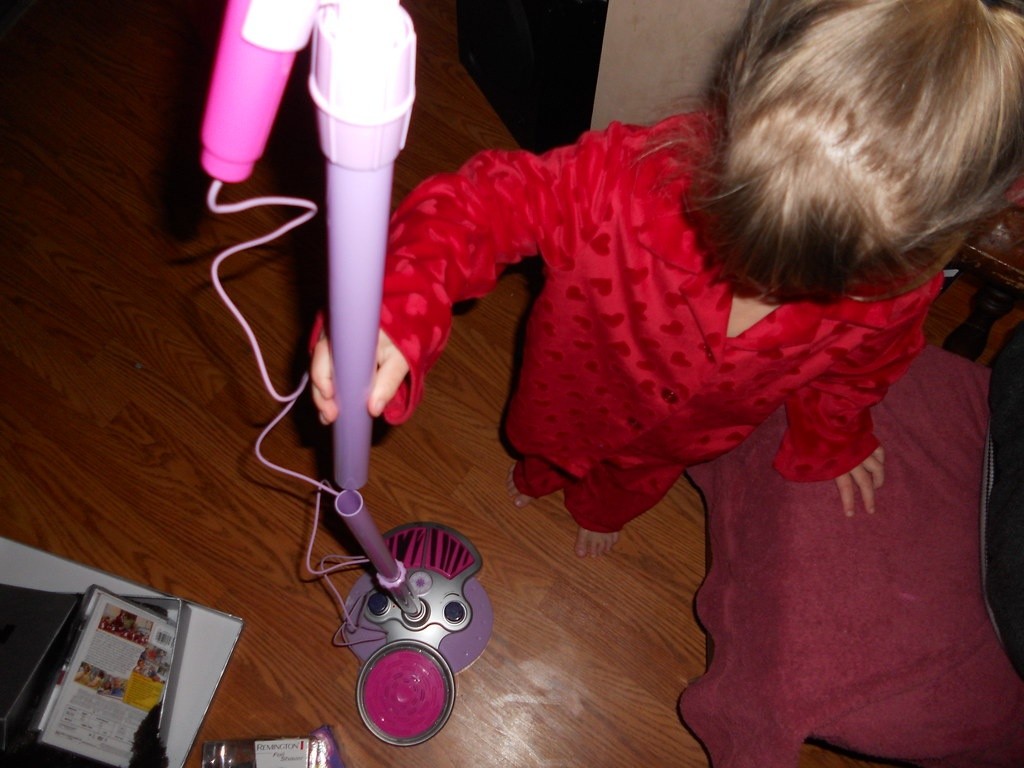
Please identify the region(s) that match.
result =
[309,0,1024,560]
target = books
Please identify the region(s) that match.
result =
[28,583,177,768]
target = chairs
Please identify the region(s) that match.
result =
[677,241,1024,768]
